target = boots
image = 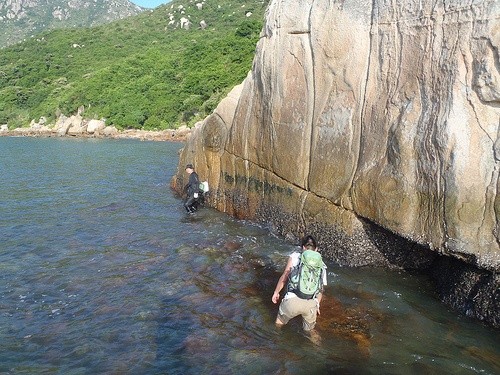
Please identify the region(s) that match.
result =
[185,205,198,215]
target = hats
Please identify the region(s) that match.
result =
[184,164,194,170]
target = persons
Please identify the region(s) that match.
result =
[182,163,205,216]
[272,234,327,343]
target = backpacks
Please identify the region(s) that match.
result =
[286,250,328,300]
